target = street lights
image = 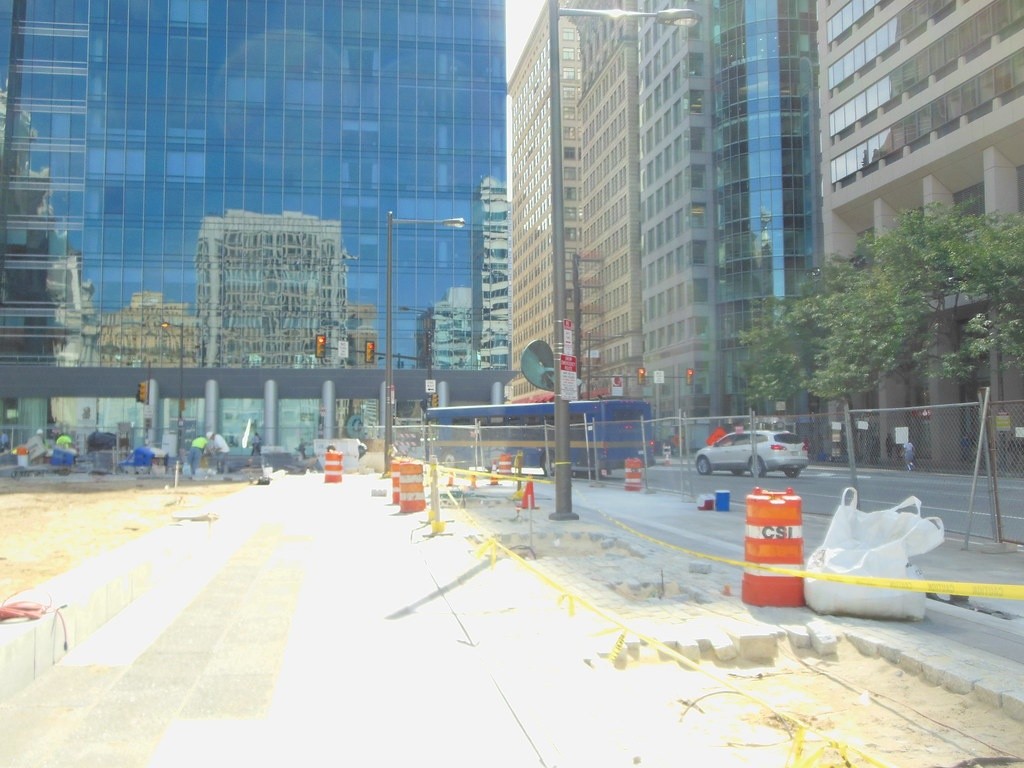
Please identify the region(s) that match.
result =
[547,4,702,520]
[162,320,184,456]
[948,276,998,433]
[383,219,469,478]
[398,307,433,379]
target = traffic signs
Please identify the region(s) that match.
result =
[425,380,436,394]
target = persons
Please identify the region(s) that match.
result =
[250,432,261,456]
[206,431,230,474]
[185,436,208,475]
[35,428,49,477]
[1,432,11,453]
[904,442,915,471]
[298,438,308,459]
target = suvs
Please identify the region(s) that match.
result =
[694,430,810,480]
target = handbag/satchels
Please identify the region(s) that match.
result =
[802,487,944,619]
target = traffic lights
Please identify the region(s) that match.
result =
[432,394,439,408]
[638,368,645,385]
[136,382,148,403]
[686,369,694,385]
[365,341,374,362]
[316,334,326,358]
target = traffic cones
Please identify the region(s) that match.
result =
[519,474,540,510]
[470,475,479,489]
[446,472,456,486]
[489,460,502,486]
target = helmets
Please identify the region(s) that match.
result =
[206,431,214,439]
[36,429,44,434]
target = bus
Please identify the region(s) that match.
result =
[426,398,657,473]
